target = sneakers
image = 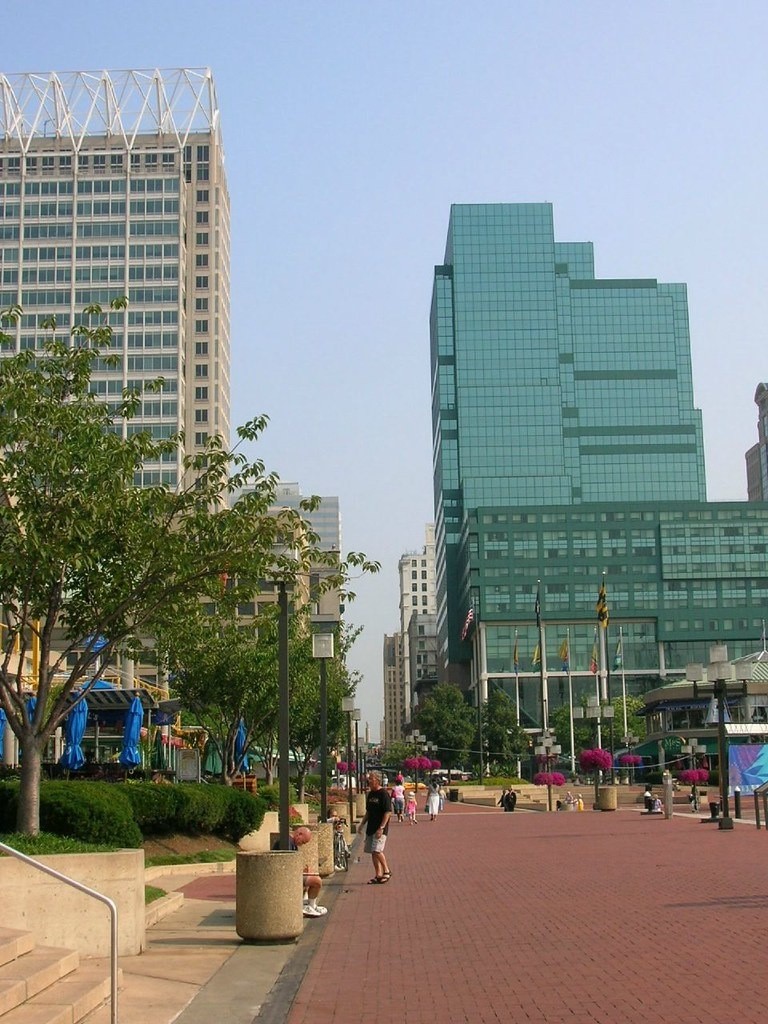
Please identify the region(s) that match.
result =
[303,904,328,915]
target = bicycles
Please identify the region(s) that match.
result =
[332,818,351,872]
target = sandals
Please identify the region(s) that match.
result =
[381,871,392,883]
[367,876,383,884]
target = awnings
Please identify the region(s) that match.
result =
[430,767,466,776]
[653,695,737,712]
[632,701,662,717]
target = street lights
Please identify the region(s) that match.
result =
[620,731,640,787]
[312,633,335,824]
[681,738,706,811]
[361,744,368,794]
[264,541,298,851]
[357,737,364,793]
[686,645,752,830]
[534,731,562,811]
[420,740,438,785]
[342,696,357,834]
[405,729,427,792]
[572,695,614,803]
[351,708,361,793]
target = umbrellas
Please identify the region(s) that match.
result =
[232,715,252,792]
[26,694,38,730]
[202,732,223,782]
[117,692,142,781]
[59,690,88,780]
[0,707,21,760]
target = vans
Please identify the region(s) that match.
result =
[332,776,356,790]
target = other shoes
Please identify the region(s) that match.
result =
[401,815,404,821]
[413,819,418,824]
[398,819,401,822]
[410,823,414,825]
[346,852,351,857]
[431,817,433,820]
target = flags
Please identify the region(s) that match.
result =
[461,607,474,640]
[612,640,622,671]
[596,583,611,627]
[591,631,599,673]
[514,637,520,674]
[531,642,542,669]
[535,587,542,628]
[559,639,569,672]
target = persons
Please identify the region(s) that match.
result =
[272,826,329,916]
[497,788,517,811]
[425,780,447,822]
[652,794,662,810]
[390,770,418,826]
[382,774,388,788]
[317,808,341,835]
[357,770,393,885]
[566,791,584,811]
